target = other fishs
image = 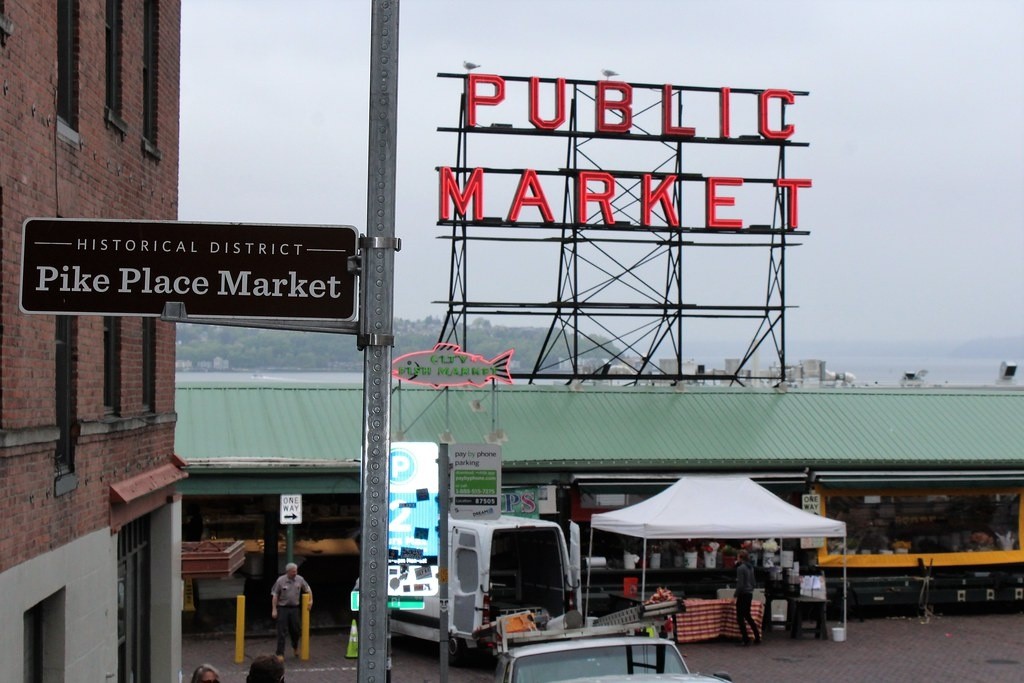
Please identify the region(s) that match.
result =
[392,343,516,389]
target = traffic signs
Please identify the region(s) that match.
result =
[280,494,303,525]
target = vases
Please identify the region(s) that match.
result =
[880,548,892,554]
[683,552,698,568]
[861,548,873,554]
[651,555,661,568]
[845,547,855,555]
[830,550,840,554]
[704,550,717,568]
[721,555,734,568]
[896,547,909,553]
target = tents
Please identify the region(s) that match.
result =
[585,477,847,628]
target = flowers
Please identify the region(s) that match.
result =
[828,534,911,550]
[648,540,770,558]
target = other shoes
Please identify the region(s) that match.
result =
[277,653,285,664]
[754,637,761,644]
[294,646,299,657]
[735,640,749,646]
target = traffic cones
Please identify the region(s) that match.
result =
[345,618,358,658]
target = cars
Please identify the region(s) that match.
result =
[494,636,732,683]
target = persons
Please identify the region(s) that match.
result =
[191,664,220,683]
[733,551,761,646]
[247,657,285,683]
[272,563,312,660]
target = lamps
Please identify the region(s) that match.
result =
[905,371,917,381]
[996,362,1017,380]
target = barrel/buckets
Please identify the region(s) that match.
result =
[623,554,638,569]
[832,627,845,641]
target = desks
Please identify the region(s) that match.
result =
[764,596,831,639]
[665,597,766,643]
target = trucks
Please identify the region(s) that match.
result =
[352,512,582,668]
